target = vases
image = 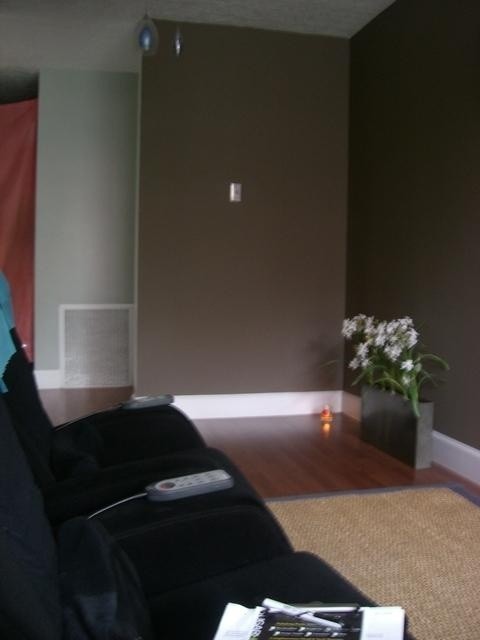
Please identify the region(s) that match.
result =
[360,384,436,471]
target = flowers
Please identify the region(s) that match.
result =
[340,311,451,419]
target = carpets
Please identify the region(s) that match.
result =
[264,480,480,640]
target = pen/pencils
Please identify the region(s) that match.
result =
[261,597,341,628]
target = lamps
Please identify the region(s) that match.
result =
[132,0,192,62]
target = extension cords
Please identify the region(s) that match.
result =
[147,469,235,502]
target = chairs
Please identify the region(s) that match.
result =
[0,268,414,639]
